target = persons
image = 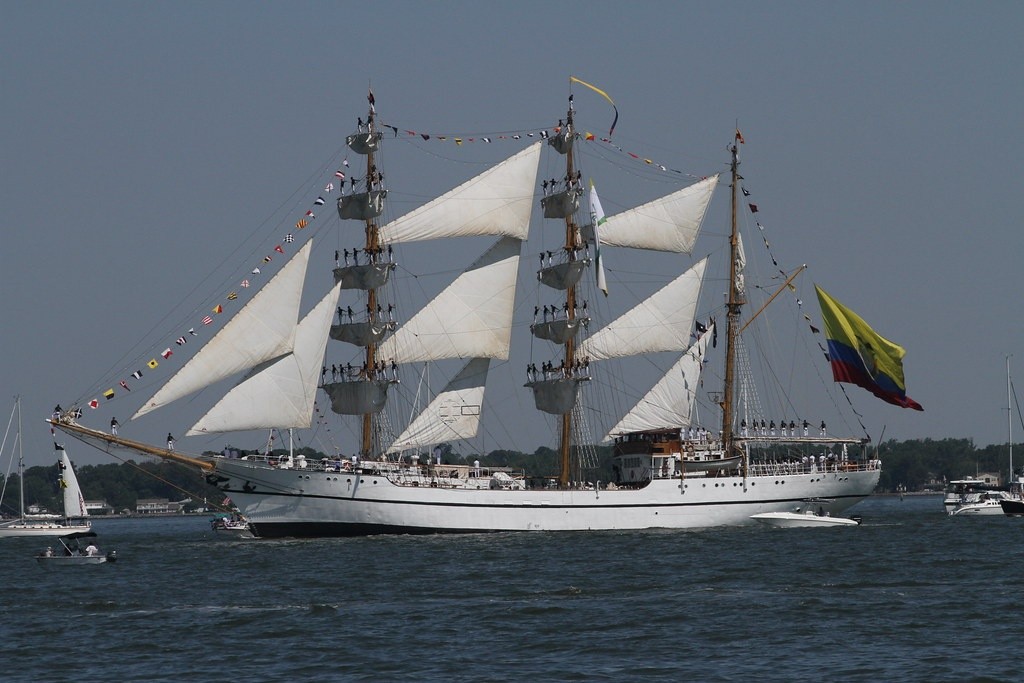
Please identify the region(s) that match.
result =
[51,404,65,423]
[167,433,175,450]
[223,447,361,473]
[110,417,120,435]
[540,170,581,197]
[434,446,442,464]
[540,241,590,270]
[473,458,480,477]
[45,541,98,557]
[531,419,883,489]
[322,117,399,385]
[528,356,590,381]
[221,512,245,528]
[818,507,826,516]
[534,300,587,323]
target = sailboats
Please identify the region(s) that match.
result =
[1,394,93,537]
[749,510,859,530]
[41,84,884,543]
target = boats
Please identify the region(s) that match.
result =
[33,550,117,566]
[943,476,1024,515]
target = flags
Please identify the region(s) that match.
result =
[73,158,349,419]
[50,423,68,489]
[382,123,564,145]
[588,177,609,296]
[585,132,924,439]
